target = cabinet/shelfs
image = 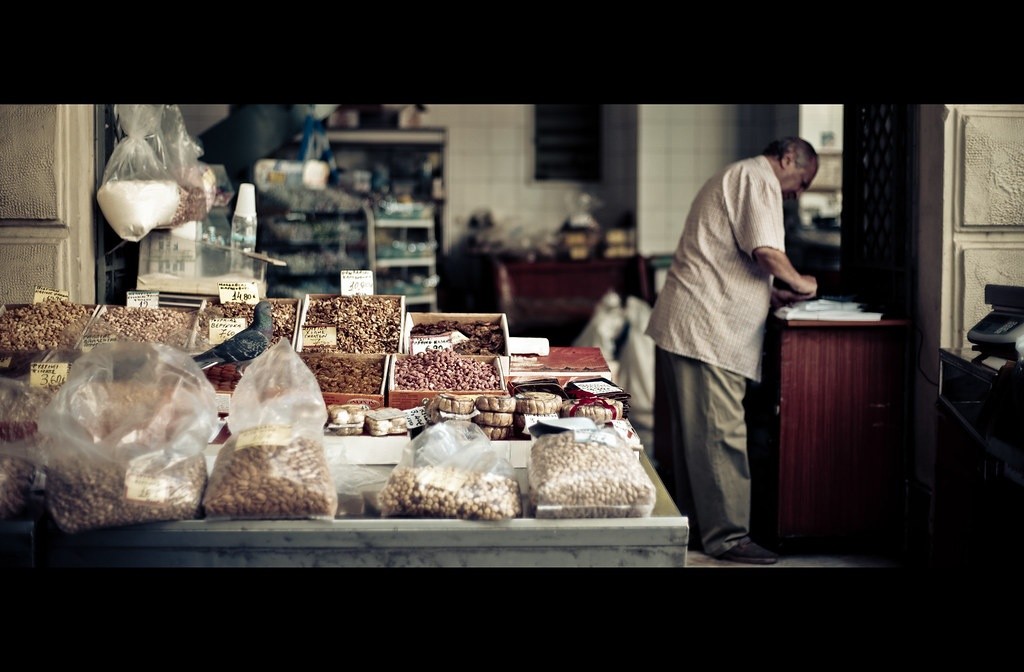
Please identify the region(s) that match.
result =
[253,131,449,302]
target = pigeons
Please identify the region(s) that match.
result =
[192,301,275,378]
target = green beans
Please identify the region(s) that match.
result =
[0,298,657,531]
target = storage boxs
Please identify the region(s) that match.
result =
[0,294,513,409]
[149,221,203,274]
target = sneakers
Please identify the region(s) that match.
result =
[722,536,779,564]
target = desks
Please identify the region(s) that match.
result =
[652,316,922,547]
[932,347,1024,549]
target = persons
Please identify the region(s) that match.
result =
[644,135,820,565]
